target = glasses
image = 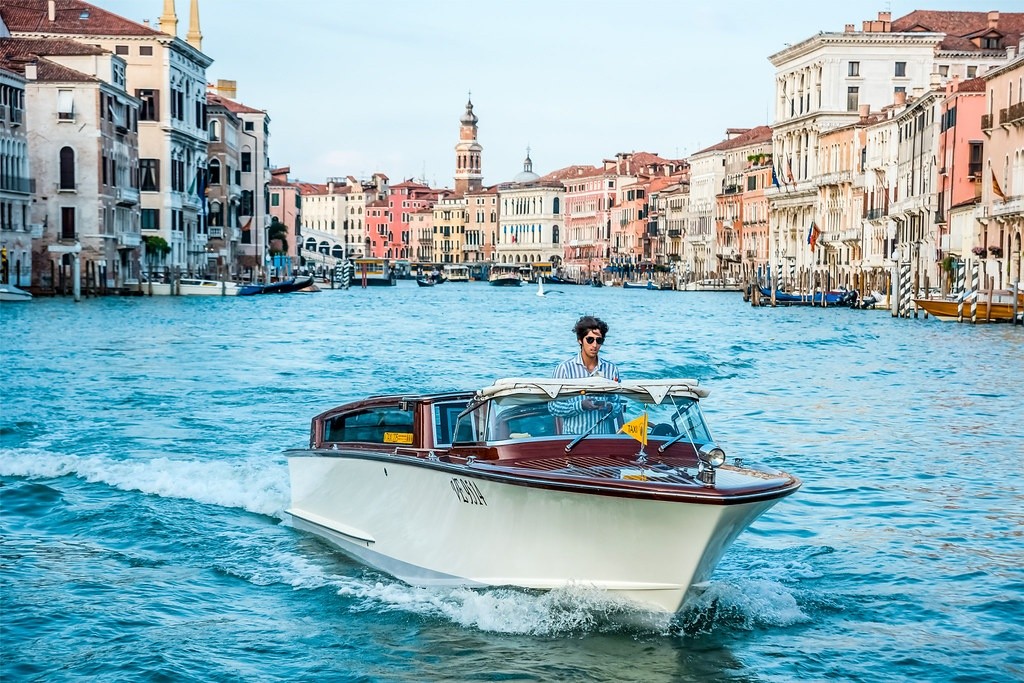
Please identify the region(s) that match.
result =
[584,337,604,345]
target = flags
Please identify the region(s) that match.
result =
[992,170,1003,197]
[772,158,797,188]
[188,174,212,209]
[808,222,820,253]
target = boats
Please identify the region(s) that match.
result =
[531,262,560,283]
[487,262,522,286]
[124,273,297,296]
[680,278,744,290]
[623,281,662,290]
[416,275,448,287]
[911,290,1024,324]
[280,376,803,613]
[287,275,315,291]
[350,258,396,286]
[759,286,857,307]
[441,264,469,282]
[0,284,32,301]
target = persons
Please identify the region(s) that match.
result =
[547,315,622,435]
